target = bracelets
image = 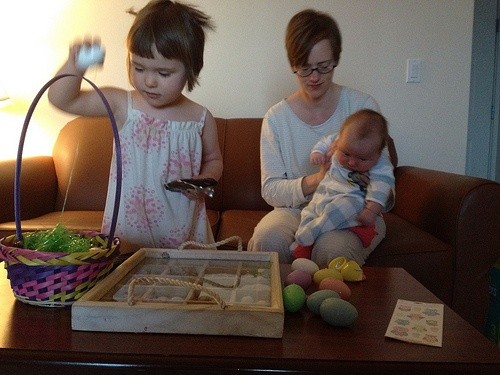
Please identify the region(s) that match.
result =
[365,205,380,216]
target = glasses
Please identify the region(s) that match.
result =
[292,64,337,77]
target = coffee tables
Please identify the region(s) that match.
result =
[0,266,500,375]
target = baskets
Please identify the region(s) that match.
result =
[0,73,123,308]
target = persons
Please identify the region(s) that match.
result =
[283,110,395,260]
[247,9,396,268]
[48,0,223,250]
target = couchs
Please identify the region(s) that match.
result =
[0,118,500,345]
